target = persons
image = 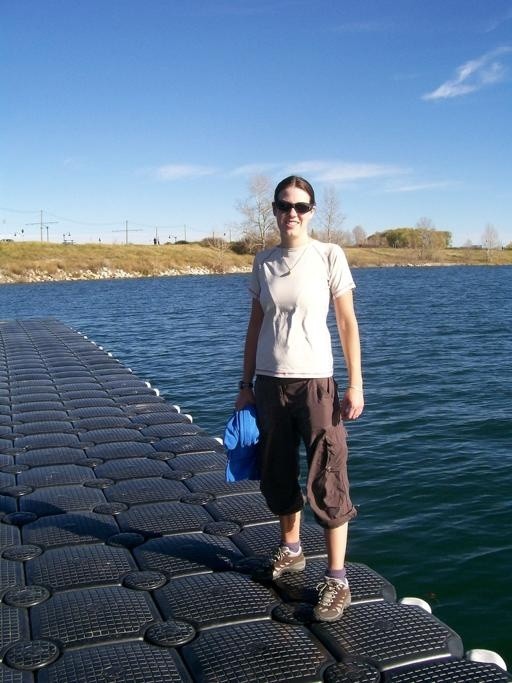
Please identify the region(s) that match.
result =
[232,176,364,622]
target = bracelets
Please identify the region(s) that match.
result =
[345,385,362,392]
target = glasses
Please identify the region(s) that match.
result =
[274,199,313,213]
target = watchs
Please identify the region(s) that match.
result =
[238,381,254,389]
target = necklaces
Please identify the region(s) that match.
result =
[277,242,316,276]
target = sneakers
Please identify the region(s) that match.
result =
[257,542,307,582]
[312,573,353,623]
